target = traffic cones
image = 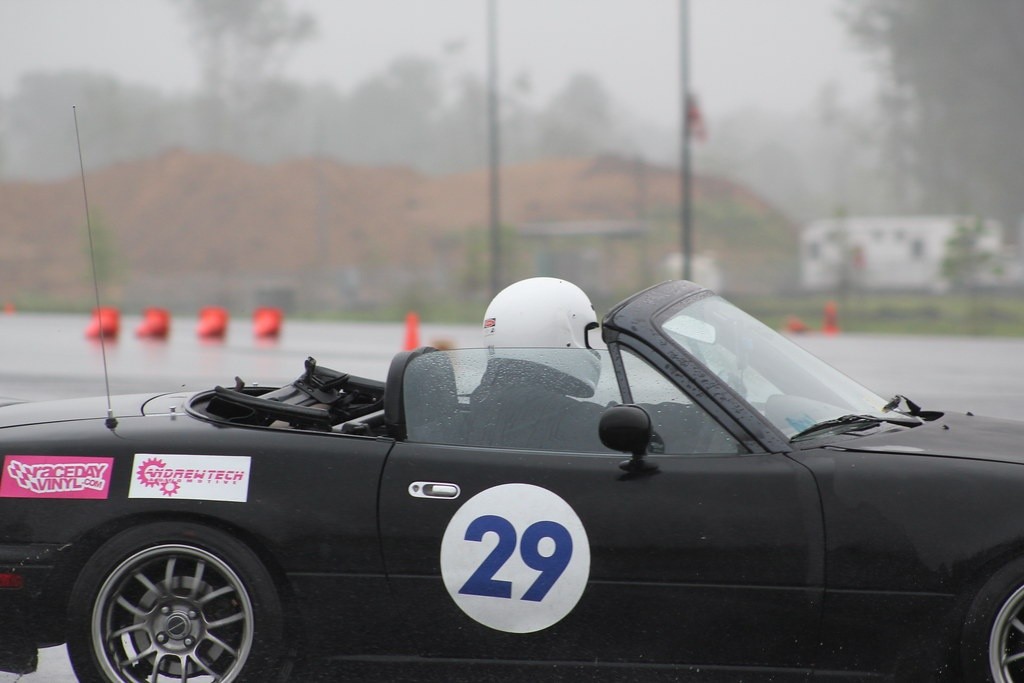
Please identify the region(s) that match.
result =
[83,305,116,340]
[135,305,169,339]
[198,303,228,340]
[784,318,808,338]
[403,310,419,353]
[824,299,842,334]
[253,305,282,338]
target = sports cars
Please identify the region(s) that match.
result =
[0,276,1024,682]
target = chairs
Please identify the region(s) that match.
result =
[414,346,470,446]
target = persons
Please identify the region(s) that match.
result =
[459,275,747,454]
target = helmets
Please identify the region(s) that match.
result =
[482,277,601,397]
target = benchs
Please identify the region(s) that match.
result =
[384,350,461,446]
